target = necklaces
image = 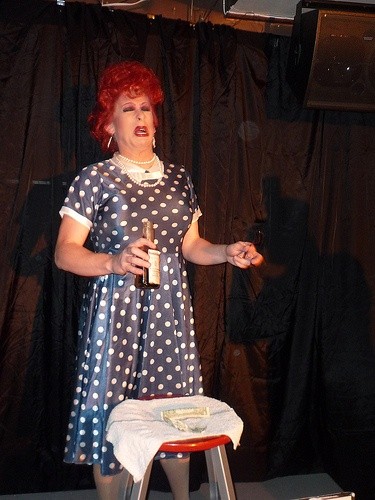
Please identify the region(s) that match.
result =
[113,150,164,187]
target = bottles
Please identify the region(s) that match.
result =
[135,221,161,289]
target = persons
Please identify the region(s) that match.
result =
[55,61,265,500]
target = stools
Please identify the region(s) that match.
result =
[119,394,236,500]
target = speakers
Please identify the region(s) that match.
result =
[293,10,375,112]
